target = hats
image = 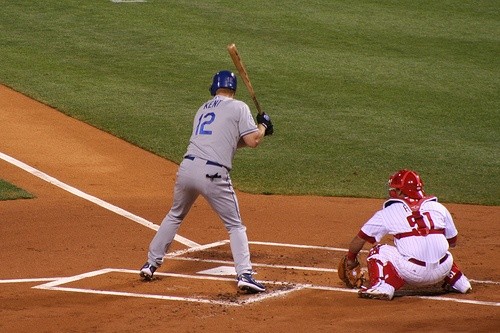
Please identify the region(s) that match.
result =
[387,168,426,200]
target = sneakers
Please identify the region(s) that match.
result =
[358,280,395,301]
[454,273,473,294]
[237,273,265,291]
[139,261,157,279]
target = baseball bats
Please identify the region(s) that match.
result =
[227,43,262,114]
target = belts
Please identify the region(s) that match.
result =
[409,253,450,268]
[183,156,222,168]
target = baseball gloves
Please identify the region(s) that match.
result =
[337,254,363,288]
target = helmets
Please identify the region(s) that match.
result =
[209,70,237,93]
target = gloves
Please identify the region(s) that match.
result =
[256,111,275,137]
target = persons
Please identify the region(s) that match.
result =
[139,70,273,292]
[338,169,472,301]
